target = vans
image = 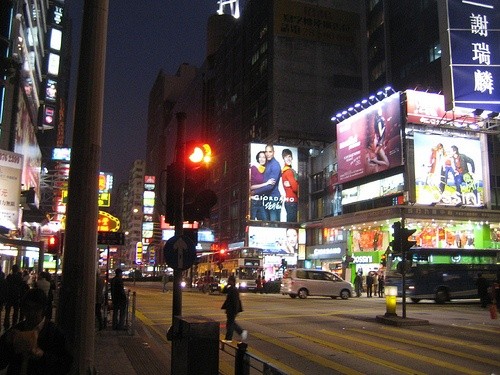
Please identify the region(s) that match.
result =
[280,269,355,300]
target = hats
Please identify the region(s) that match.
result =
[115,269,123,274]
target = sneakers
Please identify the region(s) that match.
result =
[221,339,232,343]
[240,330,247,342]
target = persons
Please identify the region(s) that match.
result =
[367,107,390,174]
[0,288,72,375]
[286,228,297,253]
[426,143,447,189]
[254,275,262,294]
[0,264,6,332]
[372,273,378,296]
[111,269,127,330]
[359,272,365,292]
[449,145,475,173]
[378,275,383,298]
[250,145,282,221]
[161,271,168,292]
[37,272,50,296]
[3,264,22,331]
[366,272,373,298]
[261,276,267,294]
[46,274,56,322]
[280,149,298,222]
[221,276,248,343]
[353,272,361,297]
[250,151,276,221]
[95,269,105,332]
[19,270,30,323]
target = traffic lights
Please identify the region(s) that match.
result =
[184,143,217,219]
[47,235,58,254]
[389,222,417,252]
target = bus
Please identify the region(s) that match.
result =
[384,248,500,304]
[179,247,264,293]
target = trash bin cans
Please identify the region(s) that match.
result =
[171,314,218,375]
